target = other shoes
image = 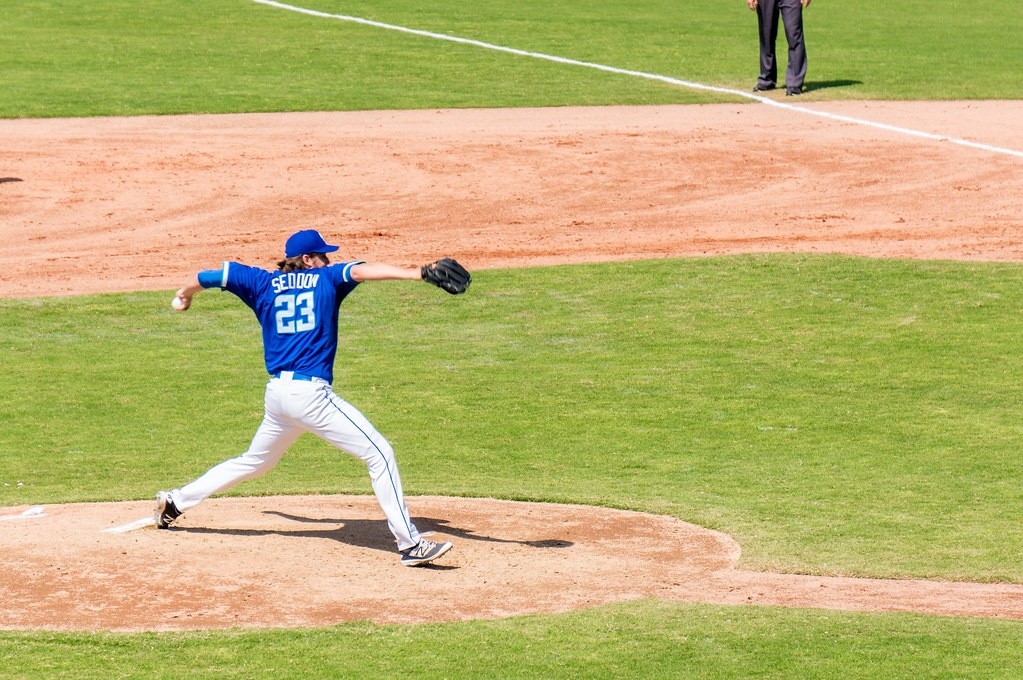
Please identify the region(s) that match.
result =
[786,86,802,96]
[753,84,776,92]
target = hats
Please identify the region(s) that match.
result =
[284,229,339,258]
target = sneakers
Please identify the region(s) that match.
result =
[154,491,183,529]
[400,538,453,566]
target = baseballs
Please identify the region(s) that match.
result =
[172,296,185,309]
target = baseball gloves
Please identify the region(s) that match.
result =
[421,257,474,296]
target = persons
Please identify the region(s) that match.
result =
[747,0,811,95]
[154,229,471,566]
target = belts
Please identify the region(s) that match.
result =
[273,370,312,381]
